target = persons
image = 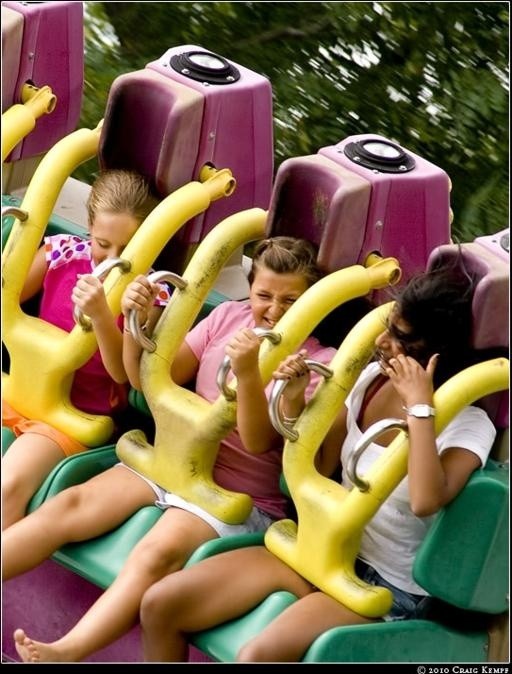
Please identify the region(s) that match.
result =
[138,233,501,667]
[2,170,178,535]
[2,236,344,664]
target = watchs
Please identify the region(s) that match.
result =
[408,403,437,418]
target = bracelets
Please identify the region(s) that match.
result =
[123,317,151,336]
[282,415,296,425]
